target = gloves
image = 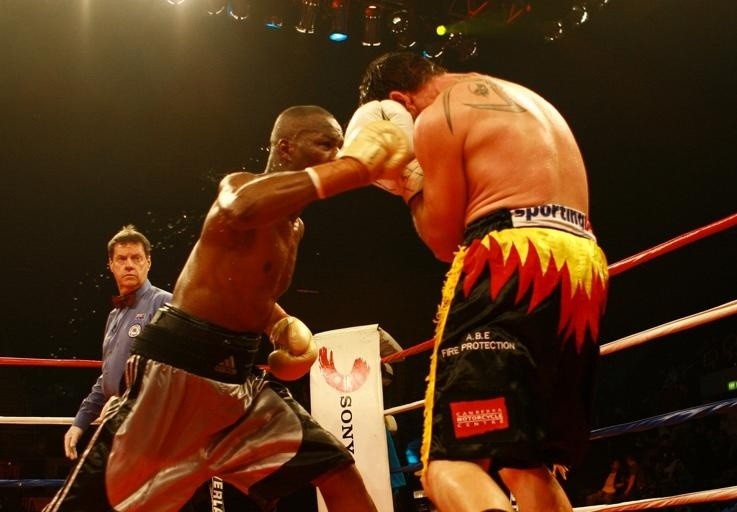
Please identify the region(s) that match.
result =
[265,313,319,382]
[64,425,85,461]
[343,119,411,181]
[337,98,423,204]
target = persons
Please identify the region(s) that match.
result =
[60,221,170,512]
[355,45,611,511]
[365,354,736,511]
[34,97,417,512]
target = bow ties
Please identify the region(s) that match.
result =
[110,292,138,309]
